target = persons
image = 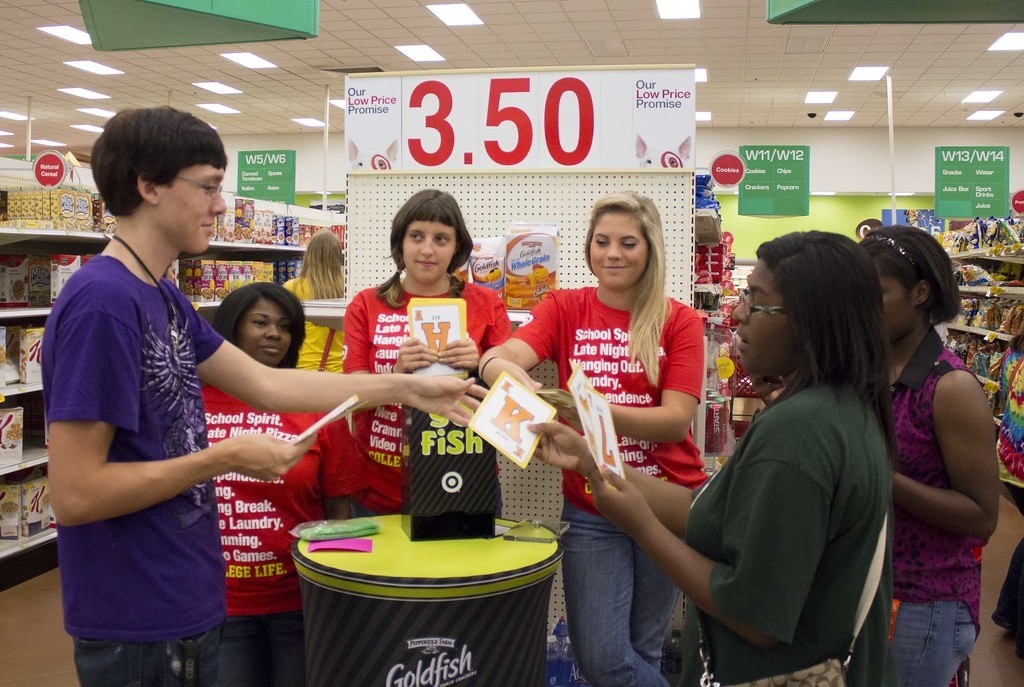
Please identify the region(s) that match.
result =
[281,229,345,373]
[525,229,892,687]
[40,104,492,687]
[200,281,325,687]
[337,188,514,518]
[476,191,709,687]
[858,224,999,687]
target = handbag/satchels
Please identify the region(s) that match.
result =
[715,658,847,687]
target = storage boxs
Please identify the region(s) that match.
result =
[3,176,343,540]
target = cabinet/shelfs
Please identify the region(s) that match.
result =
[694,197,739,337]
[1,224,299,558]
[943,243,1024,494]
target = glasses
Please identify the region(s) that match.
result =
[171,173,223,201]
[737,286,788,316]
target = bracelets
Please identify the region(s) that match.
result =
[481,356,497,380]
